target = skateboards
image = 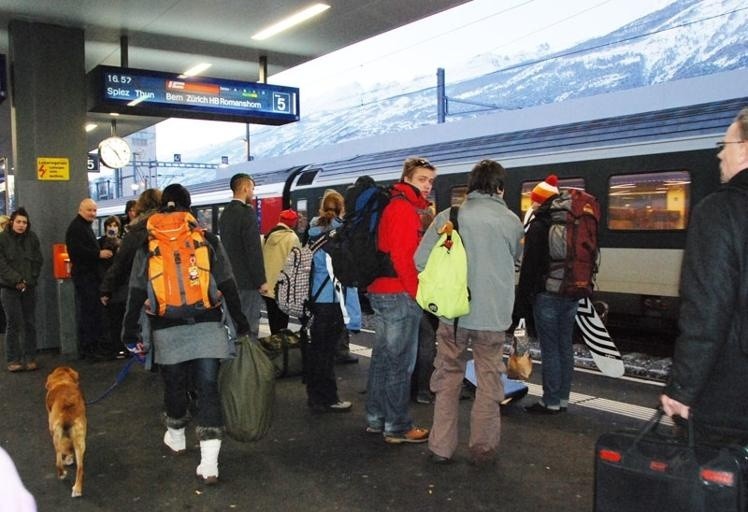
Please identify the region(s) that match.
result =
[466,359,528,400]
[576,301,626,378]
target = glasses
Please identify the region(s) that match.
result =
[714,140,746,151]
[413,158,431,166]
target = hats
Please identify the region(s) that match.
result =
[527,174,561,206]
[278,207,299,228]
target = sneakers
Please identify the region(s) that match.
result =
[415,389,438,405]
[306,397,353,411]
[382,426,431,444]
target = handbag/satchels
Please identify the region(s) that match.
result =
[592,402,748,512]
[505,317,535,382]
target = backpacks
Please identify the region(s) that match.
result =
[330,186,393,291]
[412,202,474,319]
[544,186,603,304]
[273,227,341,321]
[141,211,226,317]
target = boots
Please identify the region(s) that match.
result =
[192,437,222,484]
[161,427,189,454]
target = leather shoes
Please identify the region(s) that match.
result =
[522,402,568,414]
[6,361,39,373]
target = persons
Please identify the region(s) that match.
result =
[0,209,44,372]
[660,109,748,448]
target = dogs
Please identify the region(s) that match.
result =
[45,366,88,498]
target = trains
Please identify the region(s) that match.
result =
[92,67,747,367]
